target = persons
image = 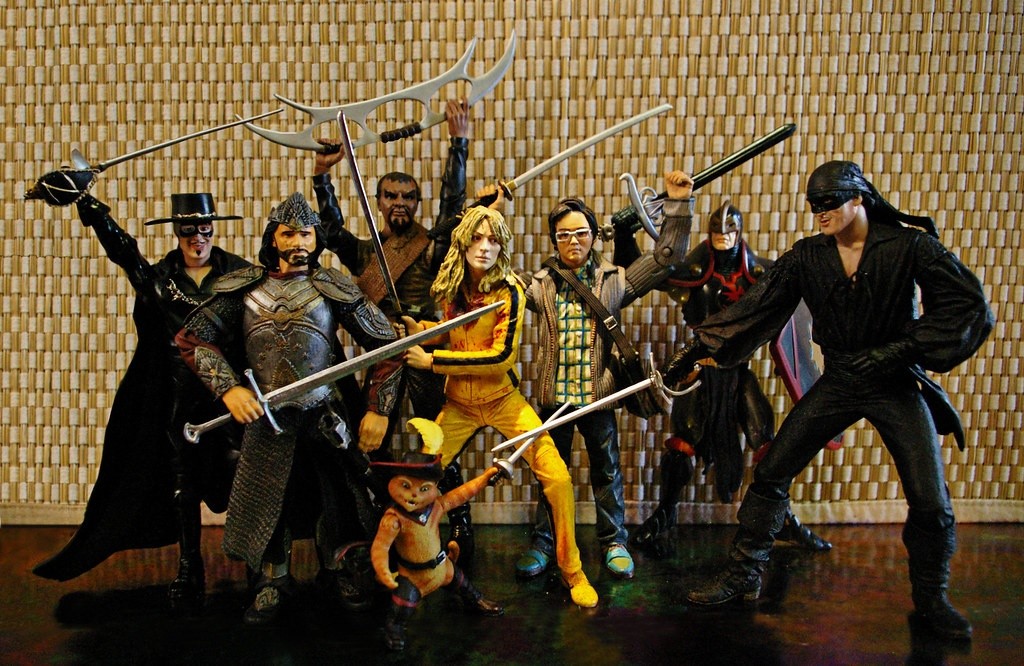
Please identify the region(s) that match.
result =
[657,160,993,641]
[33,96,833,606]
